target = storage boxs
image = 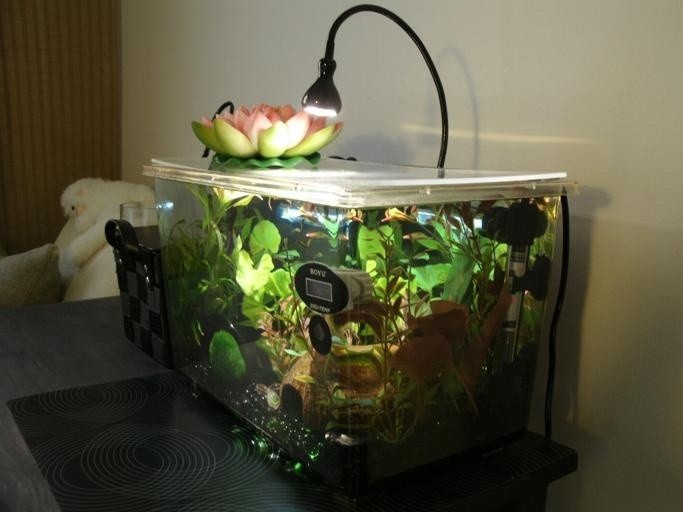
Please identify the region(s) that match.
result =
[100,217,172,355]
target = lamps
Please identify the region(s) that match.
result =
[301,4,450,169]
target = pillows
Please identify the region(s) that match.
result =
[0,243,62,307]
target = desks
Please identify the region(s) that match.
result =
[0,283,578,511]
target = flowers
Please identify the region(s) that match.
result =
[186,95,346,168]
